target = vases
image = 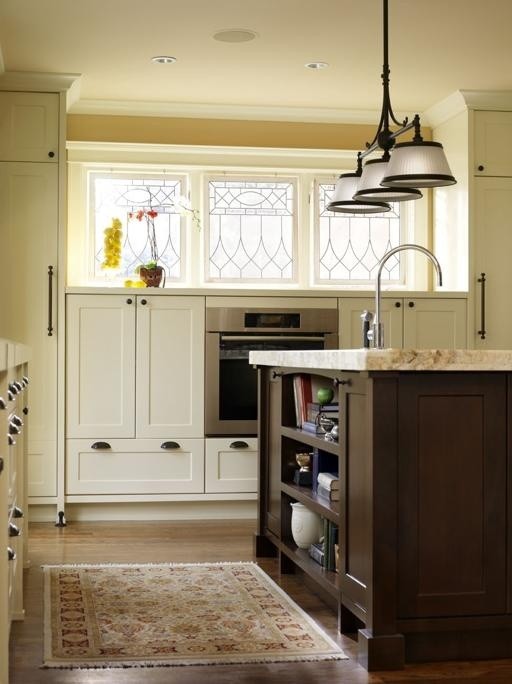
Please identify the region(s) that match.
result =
[139,267,163,288]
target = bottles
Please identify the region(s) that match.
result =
[362,310,382,347]
[321,423,339,439]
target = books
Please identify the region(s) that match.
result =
[307,409,339,419]
[309,543,323,565]
[293,376,312,427]
[317,484,341,502]
[321,518,329,570]
[301,420,327,434]
[306,400,340,413]
[329,525,339,572]
[311,377,340,401]
[316,471,341,490]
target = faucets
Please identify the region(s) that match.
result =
[368,242,444,348]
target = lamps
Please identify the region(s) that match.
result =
[327,0,457,214]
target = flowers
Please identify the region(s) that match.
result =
[99,206,166,289]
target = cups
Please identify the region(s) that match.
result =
[288,500,326,548]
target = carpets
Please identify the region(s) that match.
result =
[40,561,350,670]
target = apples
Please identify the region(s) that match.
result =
[316,386,334,405]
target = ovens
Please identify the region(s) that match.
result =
[205,307,340,439]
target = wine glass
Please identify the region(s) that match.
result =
[294,452,310,472]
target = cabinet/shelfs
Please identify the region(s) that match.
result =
[1,338,32,684]
[0,84,66,528]
[252,365,510,671]
[432,106,511,351]
